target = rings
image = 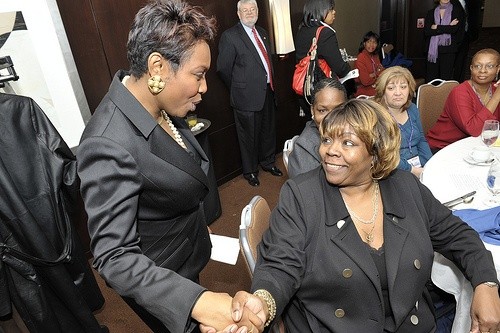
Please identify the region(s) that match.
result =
[247,325,254,333]
[497,330,500,333]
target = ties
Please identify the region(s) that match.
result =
[252,28,274,91]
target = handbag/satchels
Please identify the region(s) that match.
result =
[292,26,334,96]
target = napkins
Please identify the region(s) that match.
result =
[452,205,500,246]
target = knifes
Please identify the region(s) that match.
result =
[442,191,476,204]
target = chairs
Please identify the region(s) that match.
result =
[416,79,459,131]
[239,196,284,333]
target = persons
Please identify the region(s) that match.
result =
[353,31,386,98]
[199,100,500,333]
[295,0,359,121]
[426,49,500,155]
[217,1,283,187]
[287,77,348,179]
[373,66,434,179]
[423,0,465,86]
[76,0,264,333]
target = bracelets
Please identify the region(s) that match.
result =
[253,291,276,328]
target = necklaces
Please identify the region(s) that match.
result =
[162,109,185,149]
[472,83,491,106]
[157,112,163,125]
[339,181,378,243]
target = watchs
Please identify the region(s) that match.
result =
[482,281,499,288]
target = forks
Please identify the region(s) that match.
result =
[447,198,474,208]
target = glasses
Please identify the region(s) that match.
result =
[238,7,256,13]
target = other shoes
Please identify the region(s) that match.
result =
[100,324,110,333]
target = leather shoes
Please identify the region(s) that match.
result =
[262,166,283,177]
[243,172,259,187]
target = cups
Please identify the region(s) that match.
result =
[480,120,500,148]
[187,113,197,126]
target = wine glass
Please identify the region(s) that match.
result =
[487,161,500,203]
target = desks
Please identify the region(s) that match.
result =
[420,130,500,333]
